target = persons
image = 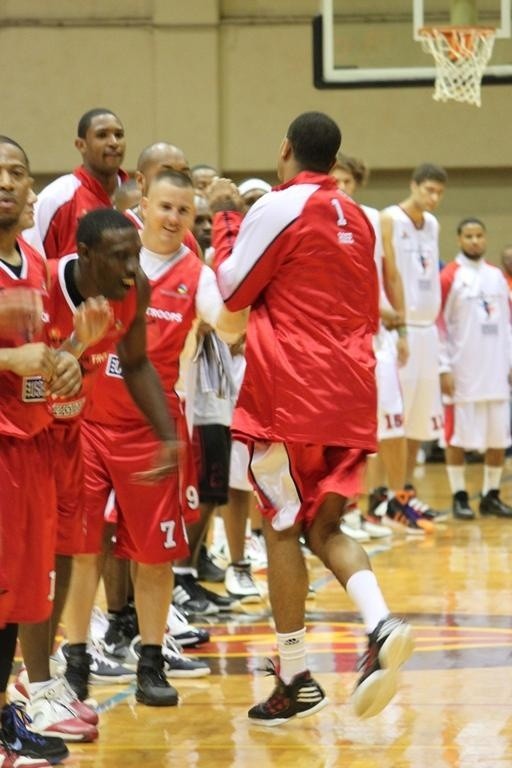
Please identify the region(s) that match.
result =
[0,108,512,768]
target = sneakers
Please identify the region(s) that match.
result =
[245,536,264,565]
[4,659,100,724]
[405,483,449,522]
[123,632,213,678]
[1,700,71,766]
[184,573,241,611]
[171,571,217,616]
[136,655,180,706]
[449,489,475,520]
[480,487,512,520]
[246,655,330,725]
[225,560,263,602]
[337,517,370,543]
[195,544,227,580]
[350,615,413,721]
[0,745,55,767]
[384,491,436,533]
[208,544,227,562]
[306,585,318,600]
[357,515,392,539]
[25,685,99,744]
[365,484,426,533]
[88,651,141,683]
[60,642,89,698]
[95,613,132,662]
[167,603,210,647]
